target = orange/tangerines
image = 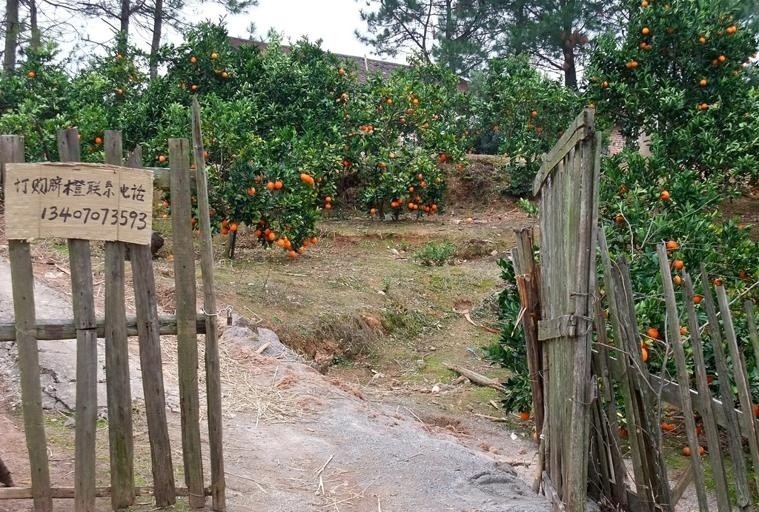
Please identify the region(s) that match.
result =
[26,48,470,262]
[518,0,759,457]
